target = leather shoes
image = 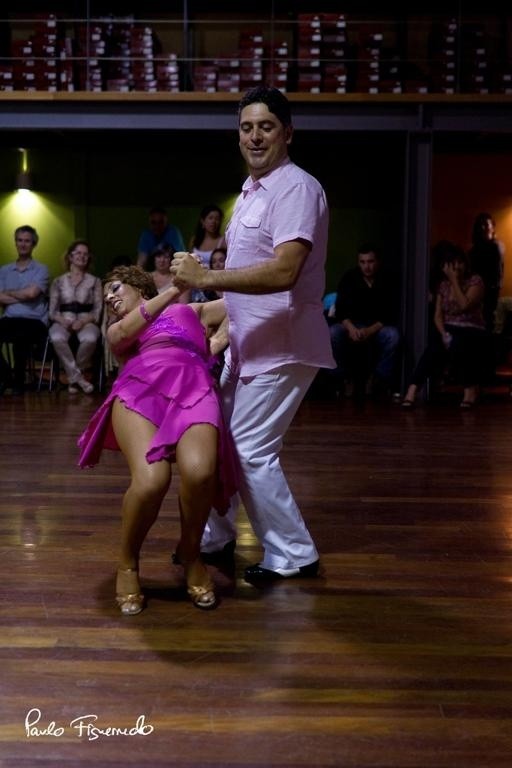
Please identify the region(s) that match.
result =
[69,387,79,394]
[84,384,94,393]
[172,539,236,560]
[245,559,319,578]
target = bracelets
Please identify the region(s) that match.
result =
[137,303,152,322]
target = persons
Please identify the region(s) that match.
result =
[0,226,51,397]
[167,87,336,587]
[187,203,228,269]
[192,248,234,390]
[469,211,502,388]
[321,243,404,405]
[147,244,175,291]
[402,247,487,408]
[76,251,231,616]
[48,240,104,394]
[135,209,188,272]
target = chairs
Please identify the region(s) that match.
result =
[0,332,103,393]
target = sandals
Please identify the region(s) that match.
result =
[460,401,473,410]
[115,567,144,615]
[402,400,413,409]
[184,566,216,607]
